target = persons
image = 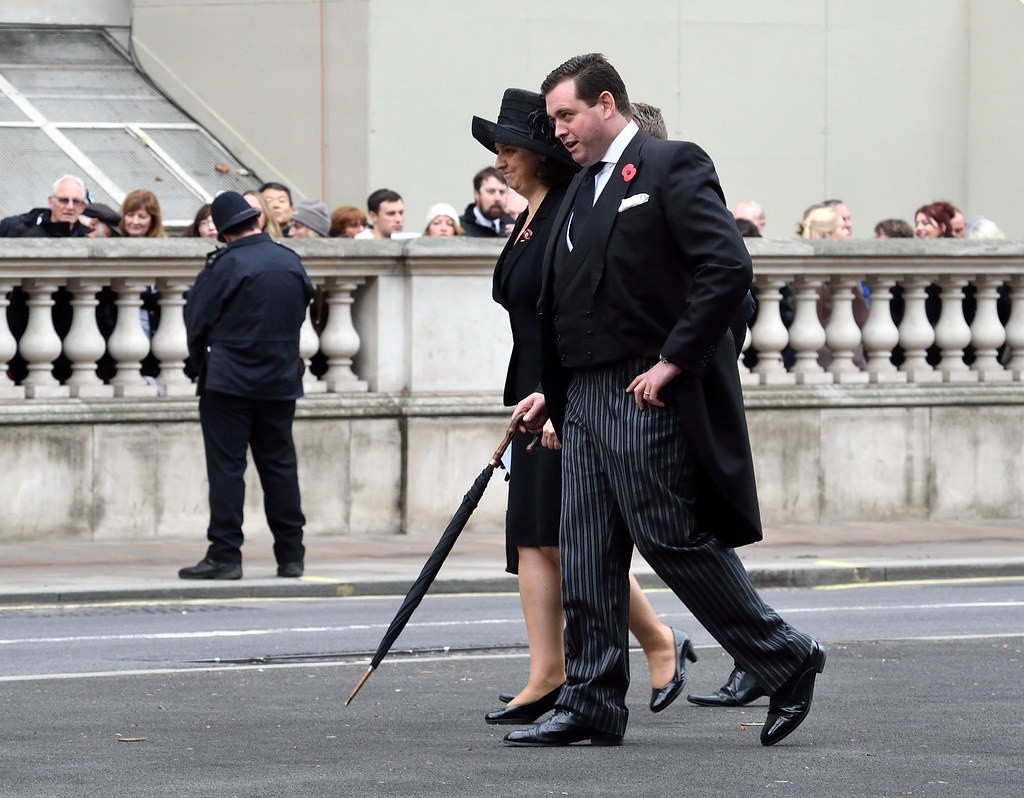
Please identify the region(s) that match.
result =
[0,167,520,387]
[472,88,699,726]
[177,190,315,580]
[732,199,1007,371]
[502,51,828,747]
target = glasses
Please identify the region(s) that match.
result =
[57,197,81,204]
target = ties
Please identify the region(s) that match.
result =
[567,160,606,245]
[492,222,496,232]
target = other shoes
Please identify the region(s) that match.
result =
[179,559,242,579]
[277,563,304,577]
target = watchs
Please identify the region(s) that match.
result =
[659,353,668,362]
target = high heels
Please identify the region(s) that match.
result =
[650,625,698,711]
[486,679,564,725]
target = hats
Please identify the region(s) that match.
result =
[290,200,332,236]
[210,190,261,242]
[472,89,564,160]
[82,202,123,236]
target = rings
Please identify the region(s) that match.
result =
[643,391,651,395]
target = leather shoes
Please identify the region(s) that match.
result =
[504,708,624,746]
[499,691,515,703]
[760,636,826,745]
[687,666,768,707]
